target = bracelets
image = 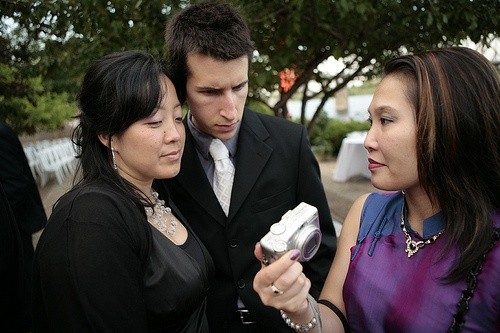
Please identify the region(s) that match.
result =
[280,310,317,333]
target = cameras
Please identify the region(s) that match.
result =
[259,201,322,264]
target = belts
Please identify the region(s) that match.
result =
[237,309,256,324]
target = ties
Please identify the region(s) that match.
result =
[208,138,236,217]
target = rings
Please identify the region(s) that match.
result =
[271,282,283,295]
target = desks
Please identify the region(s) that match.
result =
[332,132,372,184]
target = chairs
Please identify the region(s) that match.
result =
[23,136,83,187]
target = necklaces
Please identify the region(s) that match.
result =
[144,192,178,235]
[401,191,445,258]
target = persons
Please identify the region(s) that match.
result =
[0,116,46,333]
[151,2,337,333]
[254,46,500,333]
[32,49,223,333]
